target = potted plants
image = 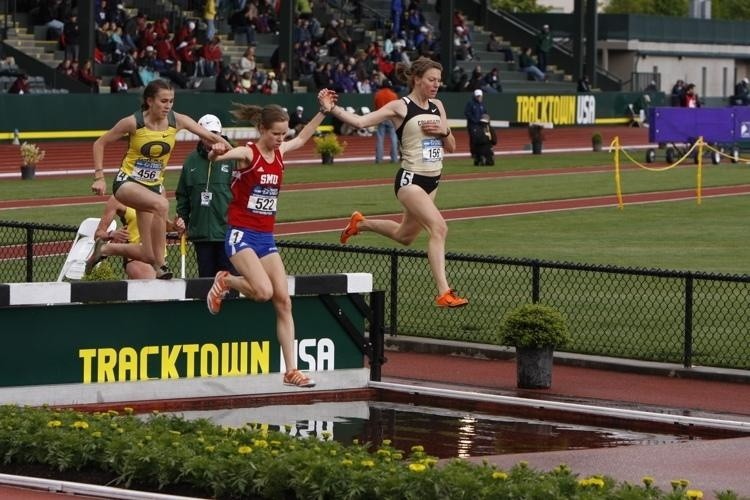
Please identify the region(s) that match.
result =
[312,132,348,165]
[592,134,602,151]
[20,142,45,179]
[499,304,576,389]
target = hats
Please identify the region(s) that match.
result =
[474,89,483,97]
[197,114,222,137]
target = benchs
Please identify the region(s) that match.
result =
[371,1,580,93]
[205,1,282,69]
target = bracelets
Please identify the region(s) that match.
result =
[440,127,450,137]
[94,169,103,172]
[107,229,113,241]
[94,176,105,179]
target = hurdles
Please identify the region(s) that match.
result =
[0,273,386,417]
[447,118,554,155]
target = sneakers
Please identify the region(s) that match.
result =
[340,211,365,244]
[206,270,231,315]
[84,237,108,276]
[157,265,173,280]
[434,289,469,307]
[282,369,316,387]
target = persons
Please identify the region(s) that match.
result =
[371,79,399,165]
[627,79,700,128]
[205,89,339,387]
[287,105,373,140]
[85,79,232,276]
[471,113,496,165]
[465,88,498,166]
[318,56,469,309]
[175,112,237,275]
[94,193,186,279]
